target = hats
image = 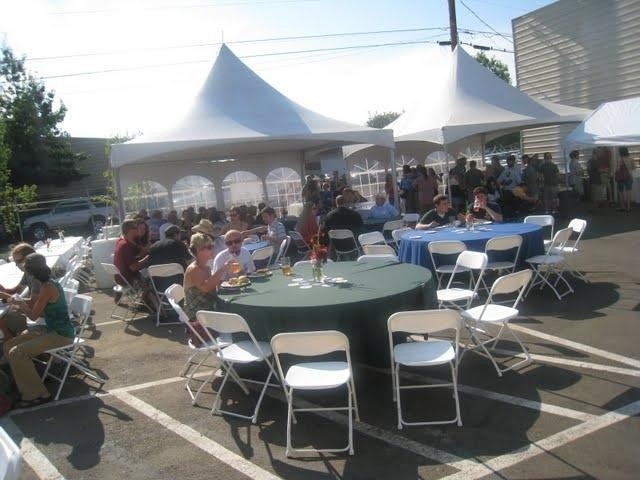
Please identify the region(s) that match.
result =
[190,219,216,237]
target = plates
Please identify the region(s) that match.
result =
[220,270,274,289]
[408,220,493,240]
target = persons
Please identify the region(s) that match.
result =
[0,242,48,366]
[372,185,540,232]
[114,196,365,340]
[303,146,637,217]
[0,253,77,409]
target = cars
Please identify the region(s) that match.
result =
[352,172,375,185]
[484,150,521,165]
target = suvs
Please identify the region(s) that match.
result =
[21,196,120,244]
[204,181,231,192]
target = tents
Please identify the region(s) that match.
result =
[343,44,594,209]
[110,44,400,228]
[563,99,640,205]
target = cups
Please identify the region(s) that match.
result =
[280,256,292,275]
[230,258,241,274]
[474,200,481,212]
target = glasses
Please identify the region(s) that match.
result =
[225,237,241,246]
